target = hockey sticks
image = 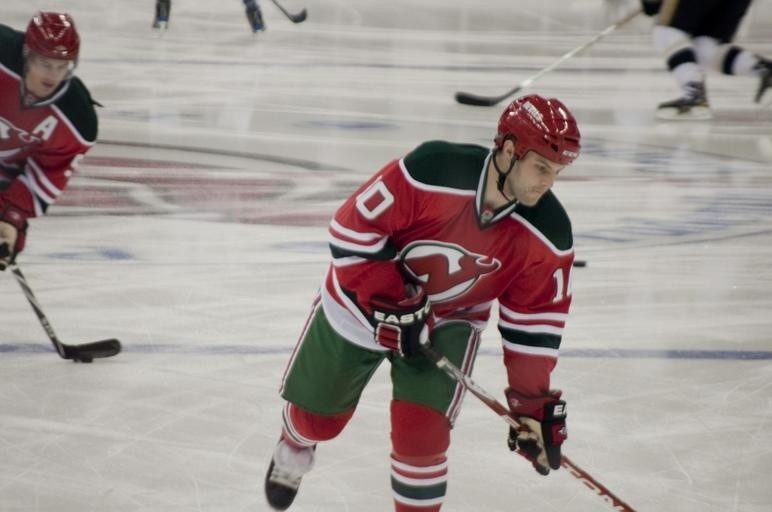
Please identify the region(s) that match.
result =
[456,1,641,106]
[273,1,309,22]
[2,241,119,360]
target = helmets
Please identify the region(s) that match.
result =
[23,13,80,59]
[492,95,582,166]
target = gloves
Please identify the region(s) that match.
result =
[503,387,566,476]
[369,287,436,357]
[0,221,18,270]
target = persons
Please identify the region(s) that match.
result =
[264,92,582,512]
[638,1,772,124]
[0,11,100,270]
[152,0,271,35]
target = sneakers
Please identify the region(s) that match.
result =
[266,440,317,510]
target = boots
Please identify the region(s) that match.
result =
[749,56,772,100]
[658,84,709,109]
